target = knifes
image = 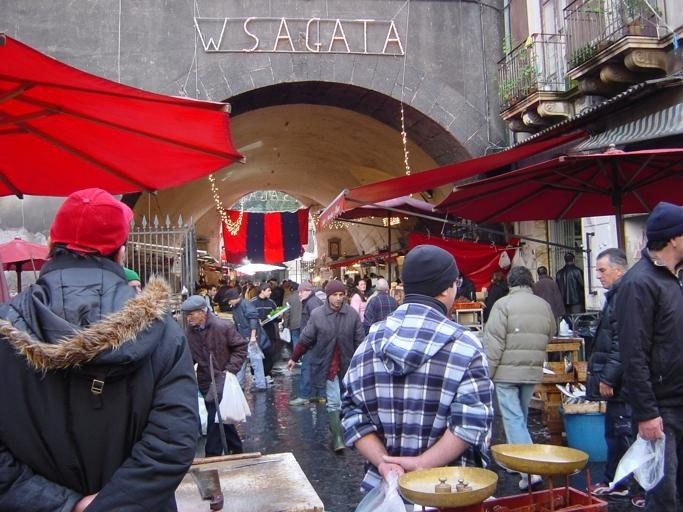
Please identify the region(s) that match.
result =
[230,457,284,468]
[189,468,223,510]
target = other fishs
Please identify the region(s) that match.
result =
[564,352,573,376]
[555,383,591,404]
[543,368,556,374]
[543,361,553,371]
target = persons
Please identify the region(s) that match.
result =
[617,201,683,512]
[221,288,266,393]
[0,188,201,512]
[349,279,367,325]
[298,281,324,335]
[585,248,646,508]
[180,295,248,456]
[250,285,289,334]
[340,245,494,512]
[229,277,269,291]
[284,281,302,365]
[204,286,217,315]
[268,279,285,307]
[556,253,585,313]
[119,265,142,296]
[455,272,476,302]
[483,267,556,488]
[287,281,366,452]
[533,266,565,337]
[478,271,509,323]
[213,279,235,314]
[363,279,400,336]
[376,275,384,280]
[346,279,357,303]
[316,288,326,304]
[322,279,329,289]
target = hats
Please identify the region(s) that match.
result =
[298,280,313,291]
[180,295,207,311]
[48,187,135,256]
[645,201,683,242]
[223,288,240,301]
[122,265,140,281]
[401,244,458,298]
[325,280,345,297]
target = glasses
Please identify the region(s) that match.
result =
[455,276,463,287]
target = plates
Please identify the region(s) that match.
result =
[489,443,589,475]
[396,466,498,508]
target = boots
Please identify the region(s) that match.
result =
[327,410,347,452]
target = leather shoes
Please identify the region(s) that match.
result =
[309,395,326,404]
[288,398,310,406]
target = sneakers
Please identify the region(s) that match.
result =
[585,481,630,497]
[266,374,274,384]
[629,494,646,508]
[518,473,543,491]
